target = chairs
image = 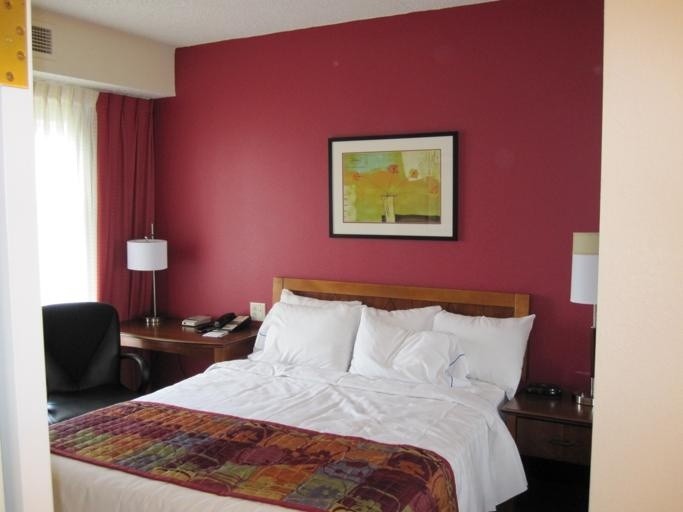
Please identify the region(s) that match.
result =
[42,302,151,427]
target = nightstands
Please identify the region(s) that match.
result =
[118,317,259,362]
[500,391,593,466]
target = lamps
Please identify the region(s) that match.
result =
[127,224,168,323]
[569,255,598,407]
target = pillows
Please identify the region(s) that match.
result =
[349,311,455,386]
[367,305,441,332]
[433,310,536,402]
[253,289,362,352]
[248,302,363,373]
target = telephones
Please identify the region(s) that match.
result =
[214,313,251,332]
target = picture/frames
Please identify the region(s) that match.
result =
[327,131,459,241]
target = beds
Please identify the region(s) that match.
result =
[48,277,531,512]
